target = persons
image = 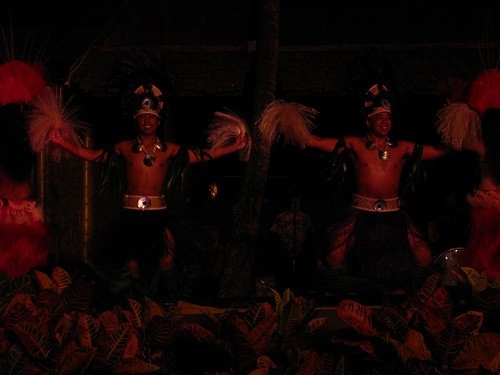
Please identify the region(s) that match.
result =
[0,172,36,215]
[298,83,452,306]
[270,196,315,253]
[51,83,247,313]
[459,134,500,282]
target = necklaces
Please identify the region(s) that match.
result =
[366,134,398,160]
[131,136,167,167]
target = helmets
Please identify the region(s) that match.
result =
[363,83,393,116]
[132,84,164,119]
